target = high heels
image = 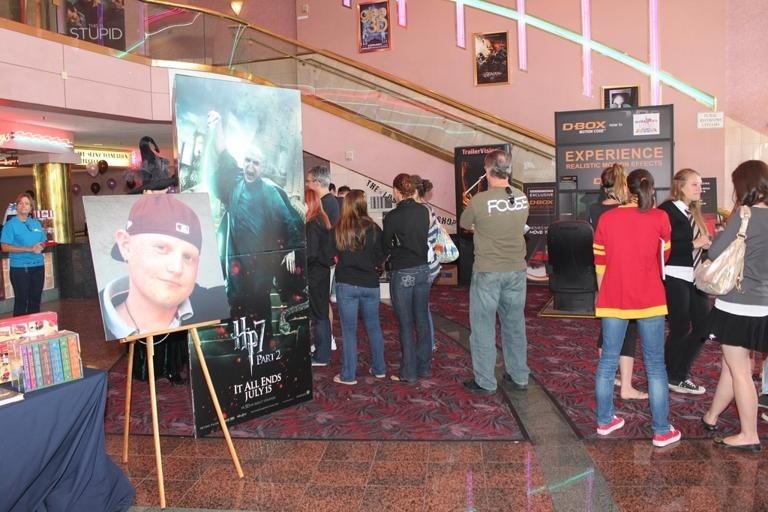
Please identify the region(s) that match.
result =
[165,371,186,386]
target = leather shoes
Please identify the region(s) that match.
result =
[464,379,496,396]
[502,373,528,392]
[701,417,718,432]
[712,437,762,453]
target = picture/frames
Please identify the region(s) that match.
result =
[356,0,390,53]
[600,83,641,109]
[473,29,511,88]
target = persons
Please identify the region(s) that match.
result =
[1,193,48,315]
[698,158,768,455]
[205,109,303,356]
[459,148,531,393]
[651,167,711,397]
[298,158,455,389]
[99,195,221,332]
[588,162,649,401]
[592,168,682,448]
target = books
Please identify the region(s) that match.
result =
[0,387,26,407]
[9,331,85,395]
[71,183,81,196]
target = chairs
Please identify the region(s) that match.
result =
[544,221,598,313]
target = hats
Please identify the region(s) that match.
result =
[125,135,176,194]
[110,194,203,263]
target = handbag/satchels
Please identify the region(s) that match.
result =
[435,216,461,264]
[694,206,752,297]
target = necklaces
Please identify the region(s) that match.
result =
[122,297,144,339]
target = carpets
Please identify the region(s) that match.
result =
[103,304,525,441]
[430,285,768,441]
[537,295,597,318]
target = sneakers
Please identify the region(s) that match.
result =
[668,380,706,394]
[596,415,625,435]
[331,340,337,351]
[311,358,329,367]
[333,374,357,385]
[369,368,386,379]
[652,424,681,447]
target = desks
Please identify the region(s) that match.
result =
[0,368,135,511]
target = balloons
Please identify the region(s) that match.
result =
[106,179,117,192]
[90,182,101,195]
[125,177,136,190]
[87,162,98,177]
[97,160,109,174]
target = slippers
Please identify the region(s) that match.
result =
[389,375,417,385]
[620,391,649,402]
